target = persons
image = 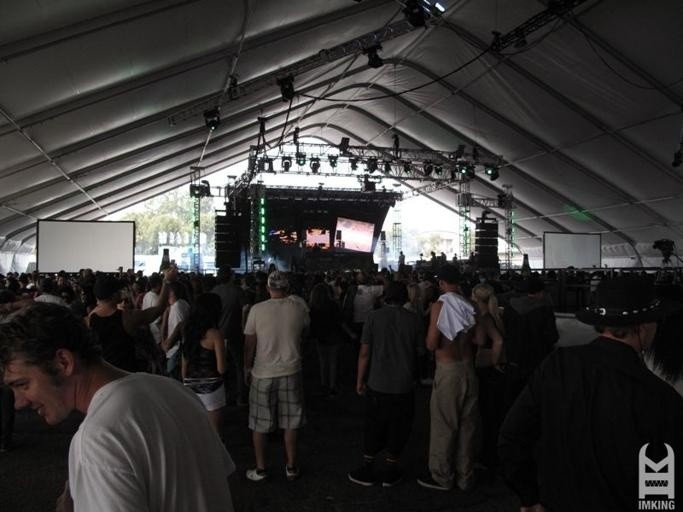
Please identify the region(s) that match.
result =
[0,301,237,512]
[416,268,483,493]
[469,283,509,477]
[180,293,230,442]
[500,285,683,511]
[242,271,310,482]
[347,280,427,489]
[0,290,34,452]
[0,249,682,410]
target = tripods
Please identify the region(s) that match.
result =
[655,259,680,286]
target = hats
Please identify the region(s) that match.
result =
[95,274,128,298]
[433,264,462,283]
[575,277,664,329]
[267,270,288,290]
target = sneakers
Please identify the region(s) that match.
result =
[285,464,301,481]
[382,473,404,487]
[348,470,375,487]
[245,467,271,485]
[416,476,451,492]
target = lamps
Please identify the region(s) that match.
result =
[405,5,425,27]
[368,46,383,68]
[310,159,319,173]
[282,157,292,171]
[281,78,294,100]
[205,111,219,131]
